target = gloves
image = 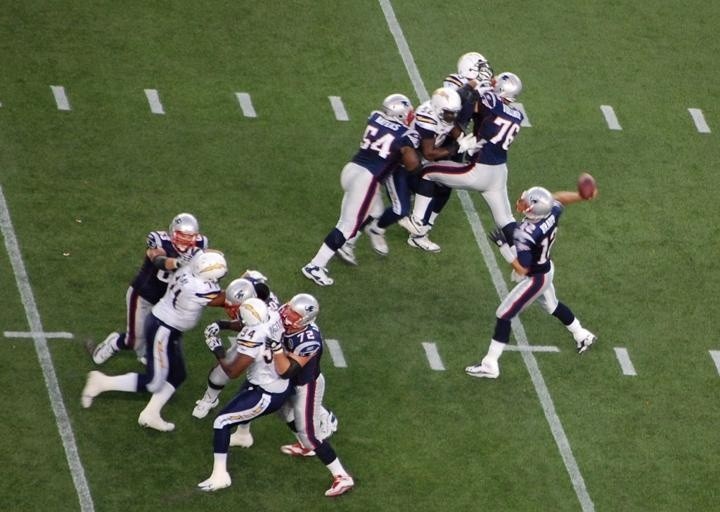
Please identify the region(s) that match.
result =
[205,336,223,352]
[265,334,282,352]
[456,132,477,153]
[176,254,192,268]
[487,224,506,248]
[204,322,220,339]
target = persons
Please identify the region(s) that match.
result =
[301,94,418,286]
[192,270,338,440]
[80,249,266,433]
[366,88,490,256]
[198,297,290,491]
[264,293,354,497]
[407,51,496,252]
[92,212,207,366]
[399,72,524,262]
[465,184,597,378]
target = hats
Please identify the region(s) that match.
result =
[225,278,257,305]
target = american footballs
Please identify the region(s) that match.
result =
[578,173,595,198]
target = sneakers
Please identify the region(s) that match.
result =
[464,362,500,379]
[337,242,359,266]
[320,423,332,442]
[301,262,334,286]
[92,331,120,365]
[229,432,254,447]
[197,472,232,491]
[324,410,337,433]
[81,370,103,408]
[191,398,220,419]
[365,220,389,256]
[280,442,316,456]
[398,216,426,236]
[407,235,441,253]
[138,411,176,432]
[577,329,598,354]
[324,475,354,497]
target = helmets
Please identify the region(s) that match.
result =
[239,270,267,283]
[496,72,522,97]
[431,87,463,113]
[168,213,200,239]
[457,51,489,79]
[382,93,411,120]
[191,249,226,281]
[520,186,554,219]
[239,298,269,326]
[287,293,320,328]
